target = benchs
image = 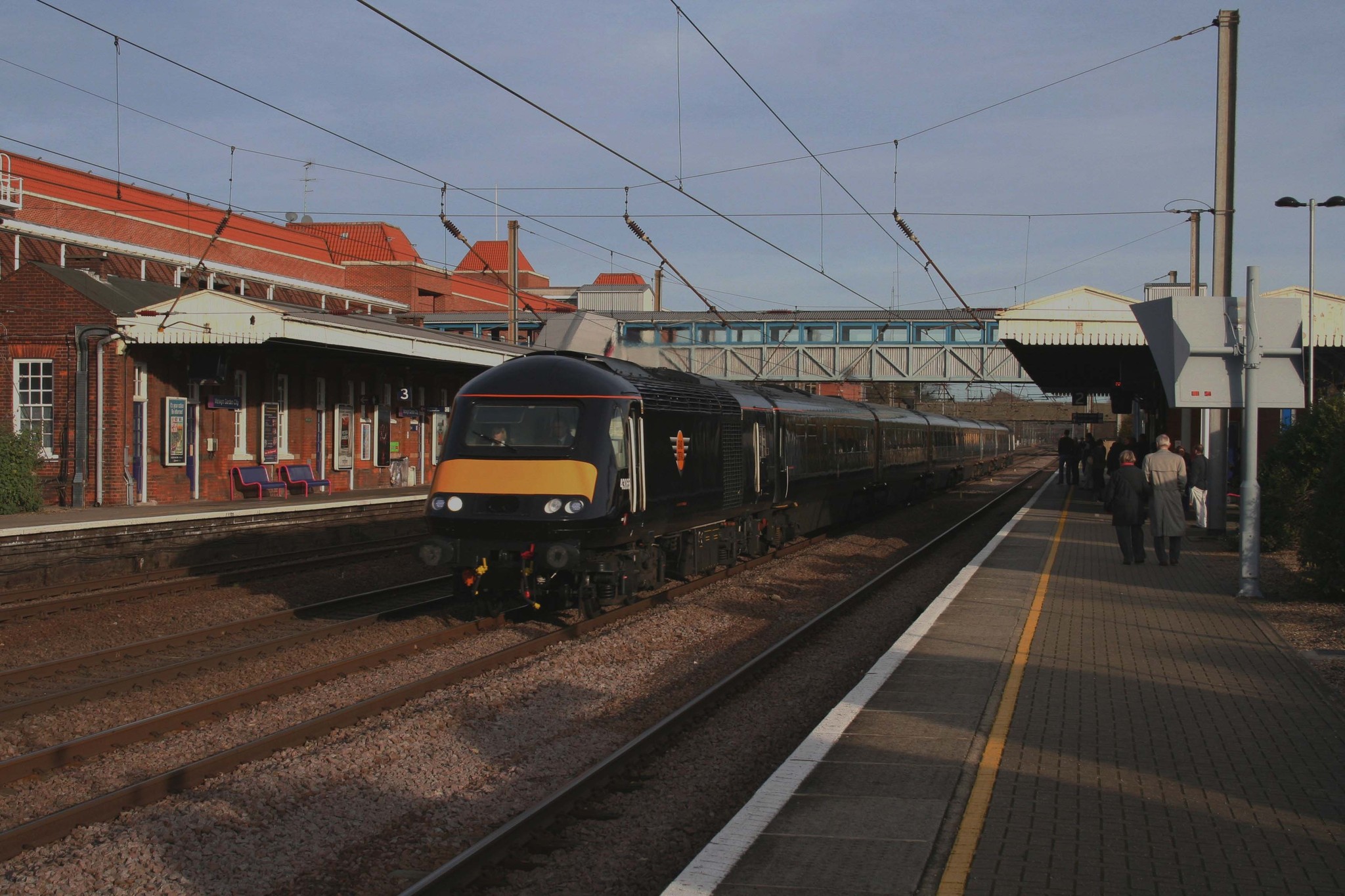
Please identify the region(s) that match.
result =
[230,465,287,501]
[279,464,332,497]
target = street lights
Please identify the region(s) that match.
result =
[1275,196,1345,414]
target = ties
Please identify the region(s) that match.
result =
[558,440,562,446]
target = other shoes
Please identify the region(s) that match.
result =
[1134,558,1144,562]
[1159,560,1168,565]
[1123,559,1131,565]
[1170,559,1178,564]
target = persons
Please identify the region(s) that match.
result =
[1105,450,1149,565]
[549,420,575,447]
[1142,434,1187,567]
[491,428,507,446]
[1058,431,1209,527]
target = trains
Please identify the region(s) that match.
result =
[424,209,1015,621]
